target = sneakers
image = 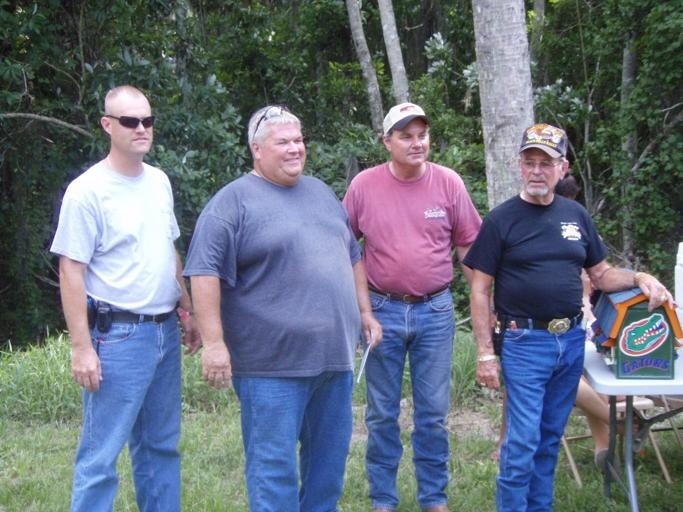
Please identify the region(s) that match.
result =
[595,448,621,484]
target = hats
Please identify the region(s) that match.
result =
[518,122,569,158]
[382,102,431,138]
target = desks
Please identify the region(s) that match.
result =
[584,339,683,511]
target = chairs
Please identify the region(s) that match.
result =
[490,390,682,488]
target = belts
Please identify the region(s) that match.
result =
[112,305,180,322]
[368,283,449,304]
[504,311,584,334]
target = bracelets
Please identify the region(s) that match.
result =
[179,311,195,320]
[476,355,495,364]
[634,270,642,288]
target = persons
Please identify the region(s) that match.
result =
[552,173,646,466]
[344,104,484,512]
[461,118,678,512]
[180,106,381,512]
[52,82,202,512]
[489,386,507,465]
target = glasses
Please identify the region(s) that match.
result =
[252,103,291,138]
[520,159,560,170]
[105,115,154,129]
